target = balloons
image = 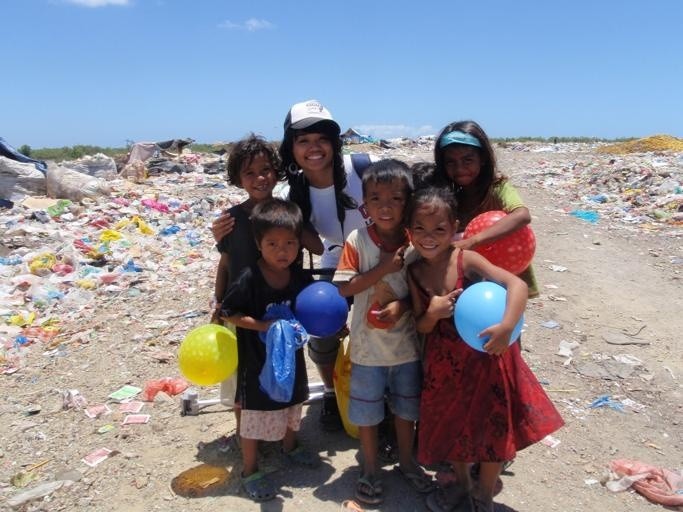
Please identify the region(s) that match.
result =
[454,281,523,356]
[178,324,239,386]
[294,282,349,338]
[463,209,536,275]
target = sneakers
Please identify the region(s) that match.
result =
[320,392,341,430]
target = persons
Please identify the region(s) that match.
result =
[213,117,397,438]
[332,160,436,504]
[435,121,538,353]
[376,162,453,465]
[402,186,564,511]
[215,137,325,451]
[218,199,350,502]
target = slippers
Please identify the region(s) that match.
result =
[240,470,275,500]
[394,464,494,512]
[355,475,384,504]
[280,442,320,468]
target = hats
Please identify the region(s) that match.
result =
[284,100,340,135]
